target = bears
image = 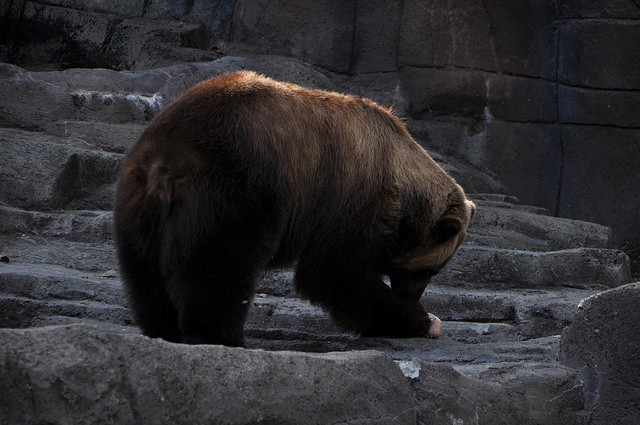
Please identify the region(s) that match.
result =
[112,69,478,350]
